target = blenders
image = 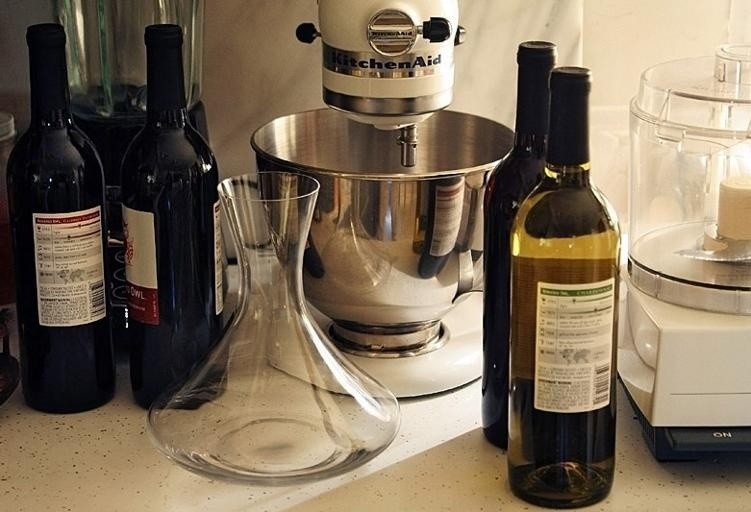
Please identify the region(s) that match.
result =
[621,44,751,468]
[249,0,516,401]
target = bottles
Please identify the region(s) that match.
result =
[49,0,211,356]
[7,23,119,417]
[504,66,620,510]
[115,22,229,411]
[482,37,559,451]
[143,171,403,488]
